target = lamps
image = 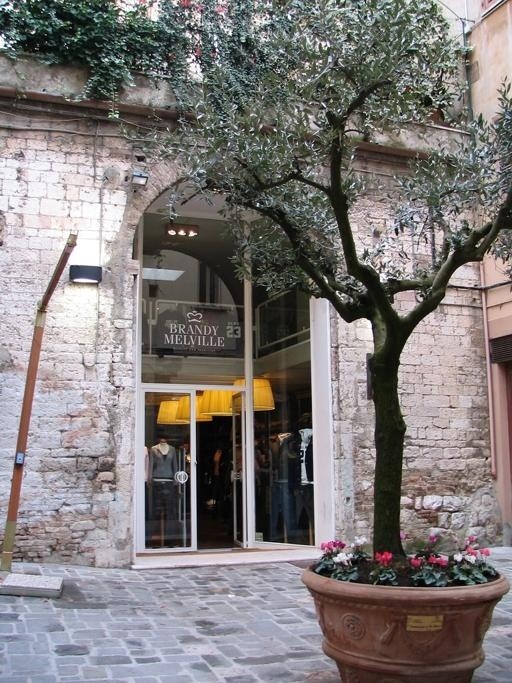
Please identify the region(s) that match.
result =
[130,173,150,185]
[163,222,202,241]
[69,264,104,286]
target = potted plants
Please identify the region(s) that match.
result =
[135,1,512,683]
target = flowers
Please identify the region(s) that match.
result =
[318,532,491,584]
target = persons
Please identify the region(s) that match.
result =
[289,426,314,531]
[210,448,227,521]
[149,436,180,546]
[265,431,298,544]
[145,445,150,545]
